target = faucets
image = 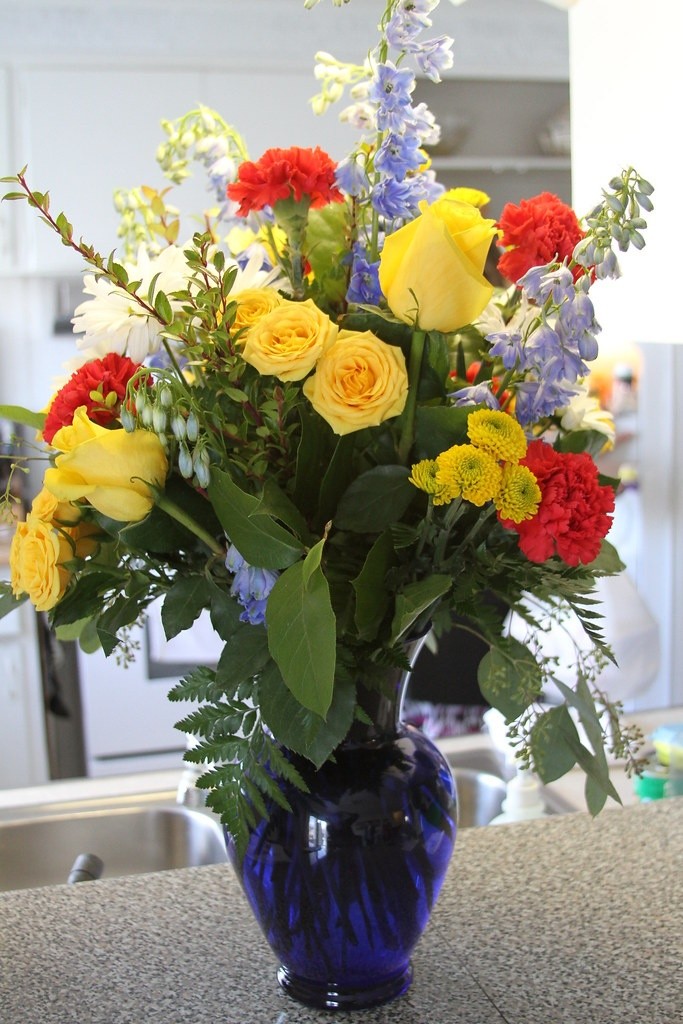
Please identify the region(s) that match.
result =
[67,853,104,884]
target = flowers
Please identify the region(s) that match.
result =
[0,0,683,850]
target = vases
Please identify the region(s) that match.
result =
[220,624,461,1012]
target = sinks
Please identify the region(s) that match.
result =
[0,787,230,892]
[441,745,580,827]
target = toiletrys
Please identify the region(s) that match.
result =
[489,757,550,824]
[176,731,207,807]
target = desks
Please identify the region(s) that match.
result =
[0,793,683,1023]
